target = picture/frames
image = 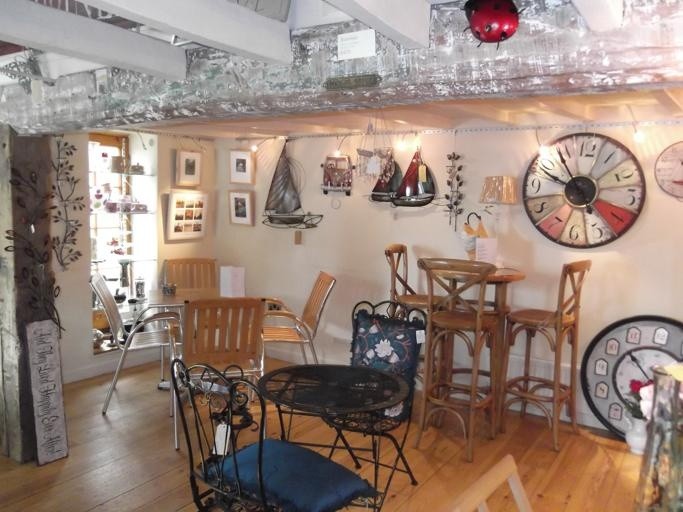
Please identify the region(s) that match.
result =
[165,188,210,243]
[228,190,255,228]
[176,149,202,187]
[228,148,256,185]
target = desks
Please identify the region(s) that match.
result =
[257,363,410,511]
[432,268,526,433]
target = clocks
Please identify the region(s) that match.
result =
[580,314,683,439]
[654,141,683,198]
[522,132,646,249]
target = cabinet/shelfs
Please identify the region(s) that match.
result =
[103,171,152,215]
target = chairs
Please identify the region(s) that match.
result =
[316,299,427,512]
[383,242,447,431]
[501,260,592,452]
[416,258,504,463]
[169,359,382,512]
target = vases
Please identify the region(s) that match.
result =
[625,414,660,459]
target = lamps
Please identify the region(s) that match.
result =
[477,175,520,264]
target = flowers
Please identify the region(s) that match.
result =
[623,379,666,419]
